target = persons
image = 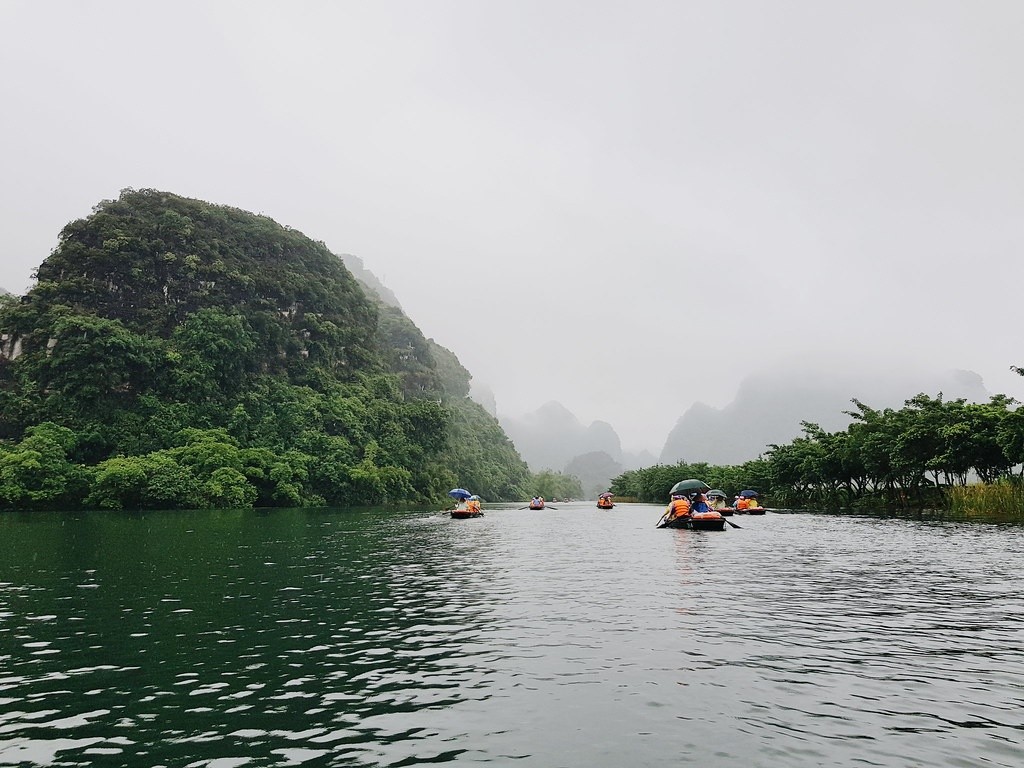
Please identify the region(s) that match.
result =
[457,497,469,511]
[530,496,544,506]
[598,497,612,505]
[708,496,726,509]
[664,493,712,524]
[733,495,758,510]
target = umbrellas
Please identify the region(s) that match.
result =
[669,479,712,504]
[706,489,727,500]
[603,492,613,498]
[448,489,472,498]
[741,490,758,497]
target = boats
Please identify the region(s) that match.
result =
[452,501,480,518]
[597,499,615,509]
[530,500,545,510]
[663,509,726,530]
[734,507,766,515]
[710,507,734,516]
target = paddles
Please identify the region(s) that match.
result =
[476,506,486,517]
[655,513,666,526]
[721,517,743,529]
[518,506,530,511]
[545,505,558,510]
[442,511,451,515]
[613,504,617,507]
[444,505,456,511]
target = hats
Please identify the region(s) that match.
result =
[740,496,744,499]
[735,496,739,498]
[692,495,706,501]
